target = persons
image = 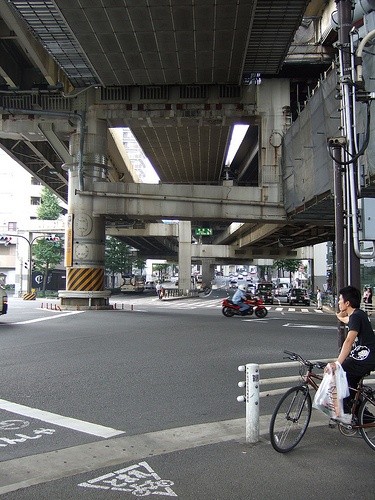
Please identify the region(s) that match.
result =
[155,280,163,297]
[322,286,375,427]
[316,289,323,310]
[362,287,372,315]
[232,284,250,314]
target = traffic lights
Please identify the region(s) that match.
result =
[0,235,12,243]
[45,237,60,241]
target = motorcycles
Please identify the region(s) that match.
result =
[222,295,269,318]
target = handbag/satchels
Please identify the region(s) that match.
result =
[334,361,350,400]
[312,363,344,423]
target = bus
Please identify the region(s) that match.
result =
[0,272,8,316]
[120,273,146,295]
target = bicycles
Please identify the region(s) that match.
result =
[269,349,375,453]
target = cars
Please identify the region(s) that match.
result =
[289,288,310,306]
[175,271,203,286]
[256,282,292,298]
[287,288,292,303]
[229,281,238,289]
[145,280,156,289]
[246,283,254,292]
[238,275,244,280]
[256,288,274,304]
[243,272,248,276]
[246,276,252,281]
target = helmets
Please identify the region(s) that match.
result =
[238,285,246,292]
